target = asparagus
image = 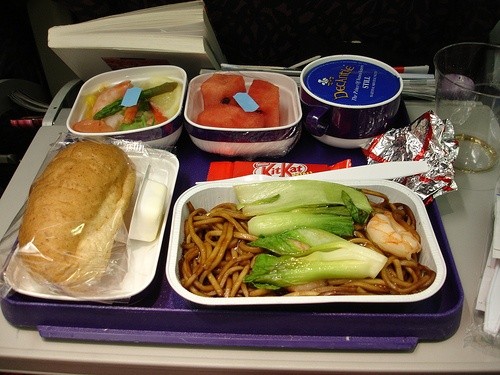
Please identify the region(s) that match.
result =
[93,81,178,120]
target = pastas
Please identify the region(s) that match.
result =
[177,188,437,298]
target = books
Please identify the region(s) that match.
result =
[199,64,437,103]
[47,1,226,83]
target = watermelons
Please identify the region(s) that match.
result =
[196,74,281,129]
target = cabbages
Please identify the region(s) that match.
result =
[234,181,389,290]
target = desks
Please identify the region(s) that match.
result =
[0,78,500,375]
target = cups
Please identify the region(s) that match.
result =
[434,42,500,171]
[300,54,402,148]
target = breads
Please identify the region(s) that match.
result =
[17,139,137,292]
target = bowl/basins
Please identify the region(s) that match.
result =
[183,71,303,157]
[65,64,189,148]
[164,176,447,306]
